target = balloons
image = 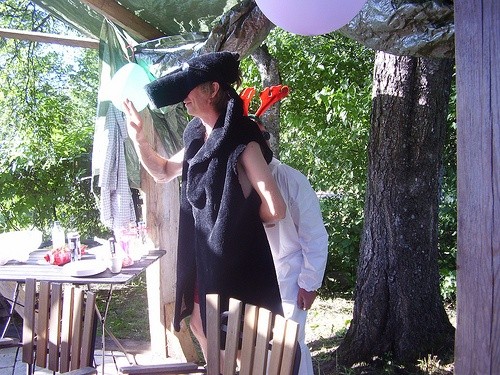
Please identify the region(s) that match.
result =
[110,63,151,113]
[255,0,366,36]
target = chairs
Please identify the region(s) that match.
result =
[0,276,98,375]
[119,293,301,375]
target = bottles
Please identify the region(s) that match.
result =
[67,228,82,261]
[52,221,65,249]
[106,217,152,274]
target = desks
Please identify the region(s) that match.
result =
[0,246,167,375]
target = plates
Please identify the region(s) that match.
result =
[64,260,108,277]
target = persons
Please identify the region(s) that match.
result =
[123,50,287,375]
[246,115,328,375]
